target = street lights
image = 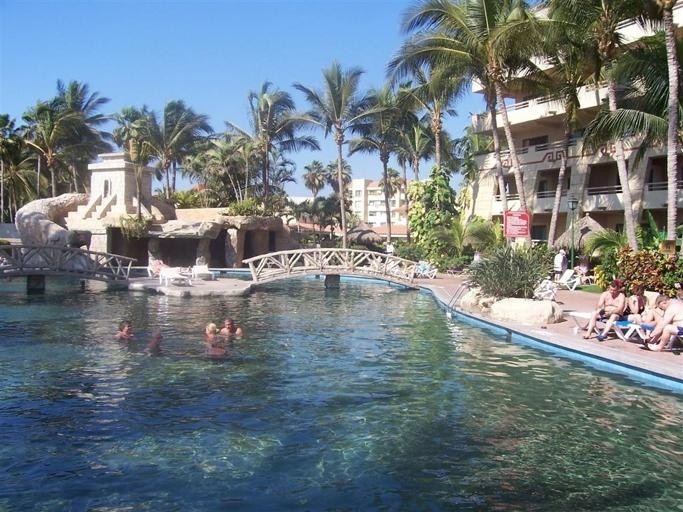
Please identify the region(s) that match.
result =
[563,194,581,271]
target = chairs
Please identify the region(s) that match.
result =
[554,268,574,291]
[410,262,473,281]
[156,263,214,286]
[565,288,682,353]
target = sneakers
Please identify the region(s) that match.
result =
[596,332,608,342]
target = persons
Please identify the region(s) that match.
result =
[384,241,395,256]
[584,279,683,352]
[574,252,589,275]
[114,317,243,356]
[554,249,564,281]
[414,258,439,279]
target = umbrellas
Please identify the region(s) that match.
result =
[553,213,605,251]
